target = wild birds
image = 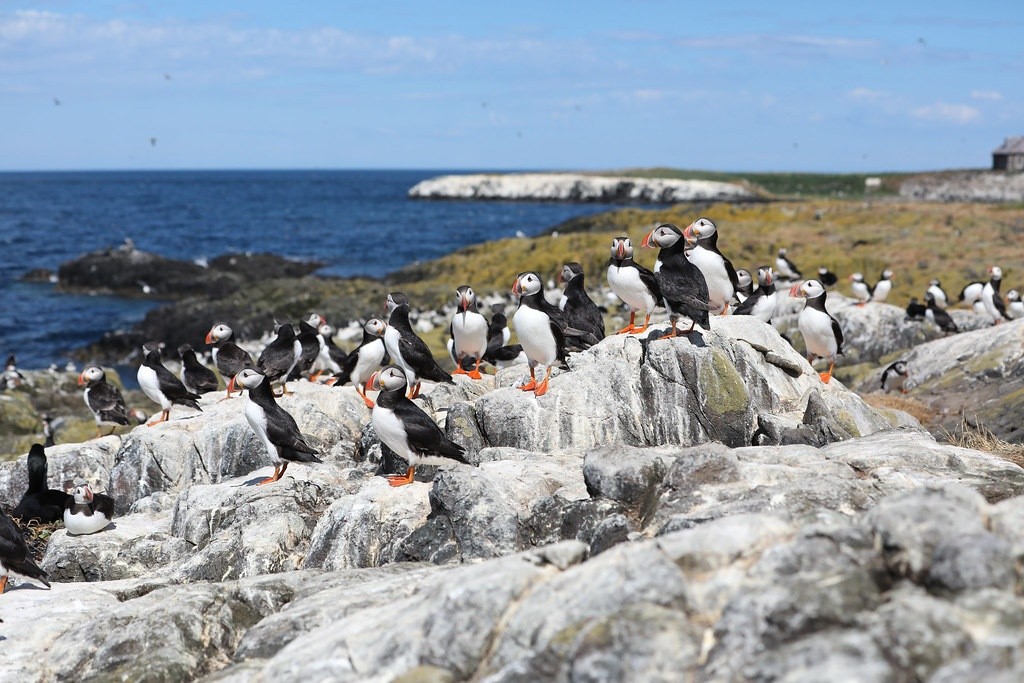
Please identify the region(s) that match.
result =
[0,218,1024,596]
